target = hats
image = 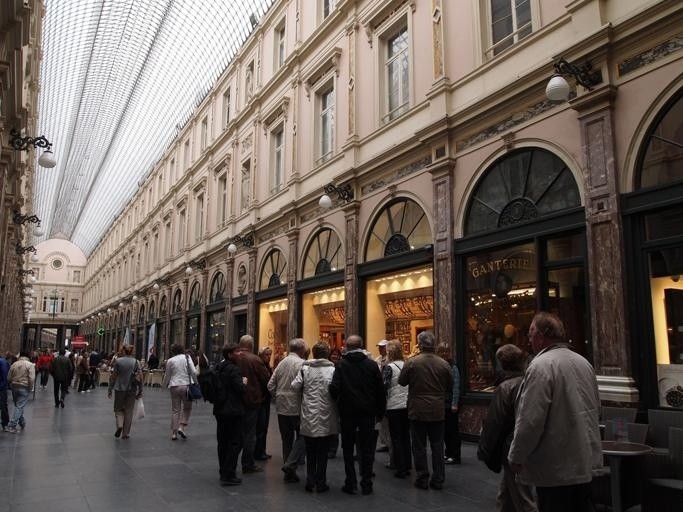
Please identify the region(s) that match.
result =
[376,339,388,347]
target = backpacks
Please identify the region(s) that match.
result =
[198,361,233,404]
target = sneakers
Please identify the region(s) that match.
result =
[315,484,330,493]
[360,477,373,495]
[39,385,42,389]
[305,486,313,493]
[44,385,47,390]
[353,455,359,461]
[86,389,91,392]
[9,426,21,434]
[172,434,178,440]
[429,480,442,490]
[281,462,300,482]
[177,427,187,439]
[81,391,85,393]
[22,422,28,430]
[341,477,358,495]
[414,481,428,490]
[4,426,8,432]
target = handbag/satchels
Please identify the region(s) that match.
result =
[185,354,202,401]
[129,359,141,383]
[337,363,384,443]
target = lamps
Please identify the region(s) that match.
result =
[153,279,170,290]
[106,305,118,312]
[81,319,86,323]
[23,305,32,309]
[91,313,97,318]
[22,284,34,294]
[228,235,253,254]
[119,298,131,307]
[319,183,351,208]
[132,290,147,300]
[546,57,594,101]
[13,213,44,237]
[77,321,81,325]
[8,129,57,168]
[185,260,206,274]
[86,317,91,321]
[16,242,39,262]
[97,310,107,316]
[18,269,36,282]
[23,298,33,303]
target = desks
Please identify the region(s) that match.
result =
[600,440,654,512]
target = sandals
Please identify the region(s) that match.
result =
[394,470,407,479]
[406,470,411,476]
[383,462,397,469]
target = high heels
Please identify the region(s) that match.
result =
[122,432,130,439]
[114,427,122,438]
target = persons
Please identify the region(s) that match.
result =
[162,345,198,440]
[0,356,9,431]
[215,342,247,485]
[7,349,36,432]
[290,343,336,492]
[374,340,389,371]
[37,349,53,389]
[255,347,273,460]
[108,344,143,439]
[328,349,342,457]
[75,347,100,393]
[146,347,158,370]
[238,335,270,471]
[398,332,455,489]
[477,344,527,512]
[269,338,309,482]
[382,339,413,478]
[64,347,76,360]
[51,348,73,408]
[507,312,603,511]
[436,342,462,464]
[328,335,386,494]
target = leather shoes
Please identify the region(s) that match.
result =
[219,474,243,485]
[375,446,389,453]
[242,463,265,474]
[444,459,454,464]
[443,455,448,460]
[55,405,59,408]
[60,398,64,408]
[253,454,272,461]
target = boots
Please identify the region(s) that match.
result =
[327,448,336,458]
[297,456,306,465]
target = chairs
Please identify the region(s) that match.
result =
[95,368,111,387]
[648,409,683,448]
[602,406,637,441]
[650,427,683,512]
[151,369,165,387]
[627,422,652,445]
[650,426,683,479]
